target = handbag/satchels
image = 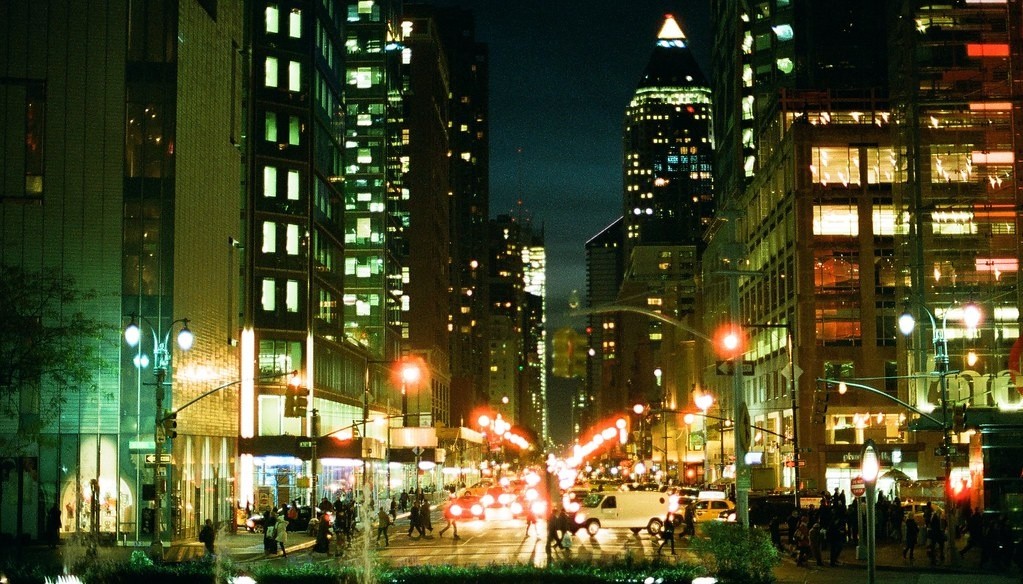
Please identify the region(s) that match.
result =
[561,534,572,548]
[794,529,804,543]
[265,526,275,538]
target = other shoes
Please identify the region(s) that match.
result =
[657,550,661,555]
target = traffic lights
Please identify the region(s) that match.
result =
[285,384,308,417]
[352,423,359,442]
[164,409,177,438]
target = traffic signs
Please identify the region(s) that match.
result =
[786,460,805,467]
[145,453,171,464]
[715,360,754,376]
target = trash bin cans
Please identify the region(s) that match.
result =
[265,517,277,554]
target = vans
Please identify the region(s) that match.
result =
[718,494,795,525]
[570,491,668,537]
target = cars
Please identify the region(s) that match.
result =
[585,480,620,492]
[246,505,334,534]
[451,472,539,520]
[676,499,735,523]
[620,482,699,511]
[899,499,944,522]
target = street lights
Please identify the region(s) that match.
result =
[724,321,800,508]
[361,357,419,502]
[898,299,980,571]
[125,314,193,561]
[860,439,880,584]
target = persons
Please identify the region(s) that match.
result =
[526,485,604,554]
[676,502,696,541]
[371,481,466,546]
[203,519,216,556]
[260,495,365,557]
[954,481,1016,575]
[657,514,678,556]
[729,487,947,567]
[46,503,63,549]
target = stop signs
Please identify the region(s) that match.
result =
[851,477,865,496]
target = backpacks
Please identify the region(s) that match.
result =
[198,529,208,542]
